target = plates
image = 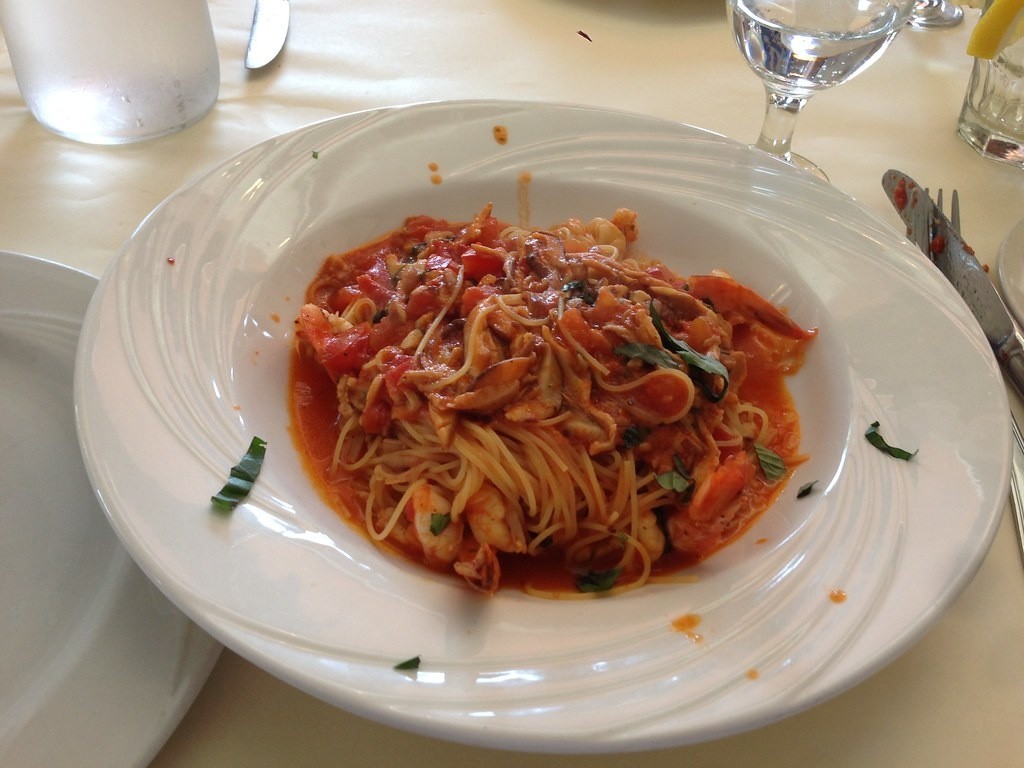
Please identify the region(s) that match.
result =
[0,250,225,768]
[74,100,1014,754]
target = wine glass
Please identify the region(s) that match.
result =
[908,0,964,28]
[725,0,918,186]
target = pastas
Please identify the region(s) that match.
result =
[326,237,776,601]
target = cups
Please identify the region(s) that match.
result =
[0,0,220,145]
[956,0,1024,166]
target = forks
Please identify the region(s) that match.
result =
[905,188,1024,567]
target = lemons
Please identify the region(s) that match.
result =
[966,0,1024,60]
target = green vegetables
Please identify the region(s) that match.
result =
[210,296,922,674]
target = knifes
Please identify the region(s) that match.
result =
[882,168,1024,401]
[245,0,290,70]
